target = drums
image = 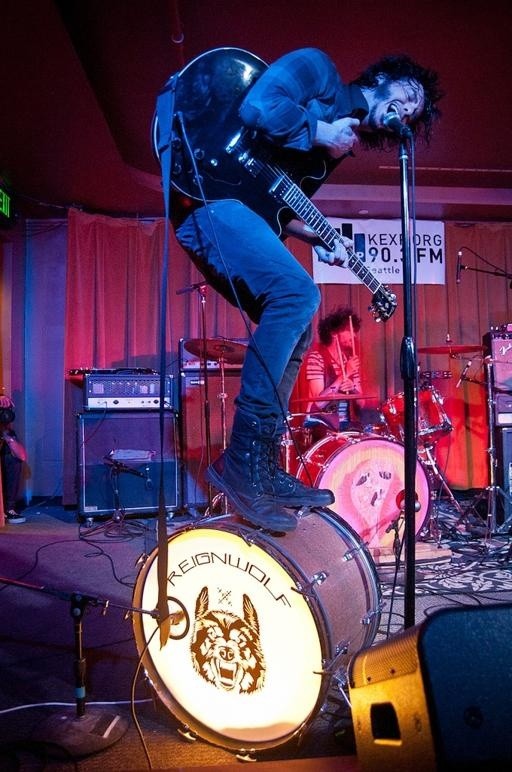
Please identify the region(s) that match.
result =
[376,385,452,453]
[297,431,431,556]
[131,498,381,757]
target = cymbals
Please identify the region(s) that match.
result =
[289,394,377,401]
[417,345,487,354]
[184,338,247,364]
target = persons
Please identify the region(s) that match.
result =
[304,304,366,425]
[170,45,449,536]
[0,392,30,526]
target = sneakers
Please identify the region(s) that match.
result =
[5,510,26,524]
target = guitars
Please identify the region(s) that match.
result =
[152,47,398,322]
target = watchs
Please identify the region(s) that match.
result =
[7,437,15,446]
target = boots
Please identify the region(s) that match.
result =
[205,408,336,532]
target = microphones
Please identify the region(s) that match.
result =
[176,280,208,295]
[456,250,462,284]
[381,112,414,139]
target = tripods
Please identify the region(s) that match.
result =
[203,354,229,520]
[77,468,155,540]
[421,443,475,548]
[452,353,511,556]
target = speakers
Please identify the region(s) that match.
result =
[344,601,512,771]
[493,429,512,531]
[483,330,512,427]
